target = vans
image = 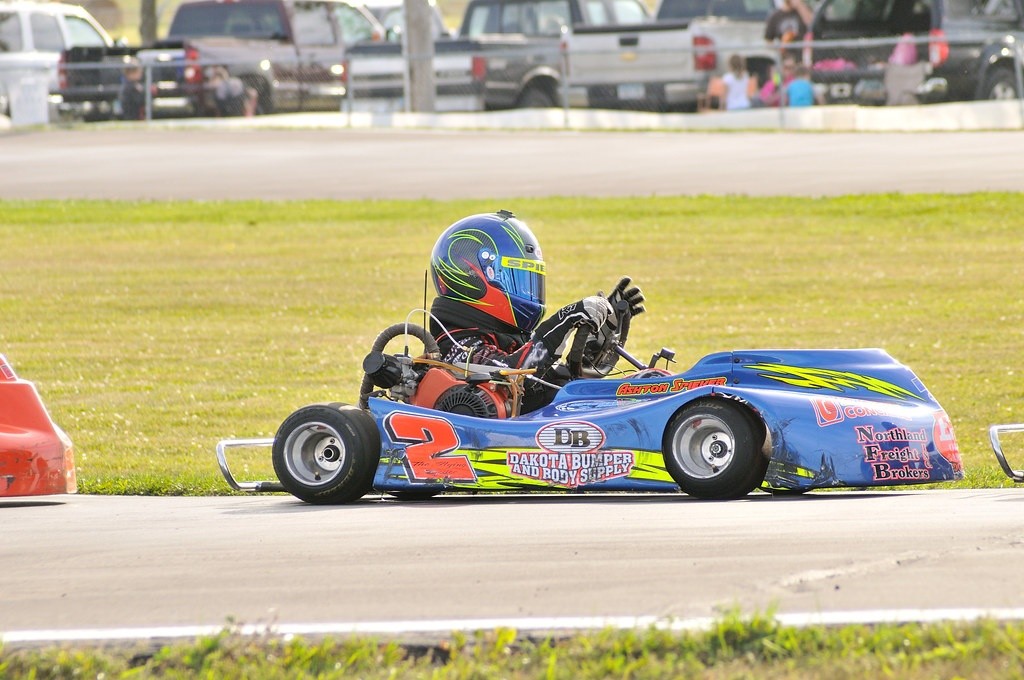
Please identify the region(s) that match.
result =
[0,1,126,118]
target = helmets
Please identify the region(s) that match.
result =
[430,210,547,333]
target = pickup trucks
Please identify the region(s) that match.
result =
[58,1,403,118]
[344,0,652,111]
[561,1,813,113]
[779,1,1023,99]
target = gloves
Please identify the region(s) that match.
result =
[534,297,613,358]
[592,277,645,345]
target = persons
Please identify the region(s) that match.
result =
[428,209,646,417]
[689,34,963,114]
[110,56,265,120]
